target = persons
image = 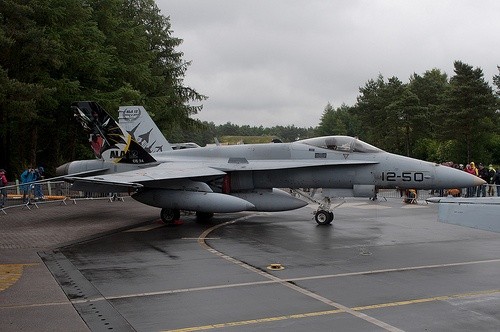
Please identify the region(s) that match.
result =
[0,169,7,207]
[34,167,45,202]
[20,169,40,203]
[398,161,500,203]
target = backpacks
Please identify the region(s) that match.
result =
[0,177,4,187]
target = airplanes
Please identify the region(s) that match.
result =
[58,101,488,227]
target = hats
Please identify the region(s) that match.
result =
[0,169,6,173]
[479,163,484,166]
[39,167,44,172]
